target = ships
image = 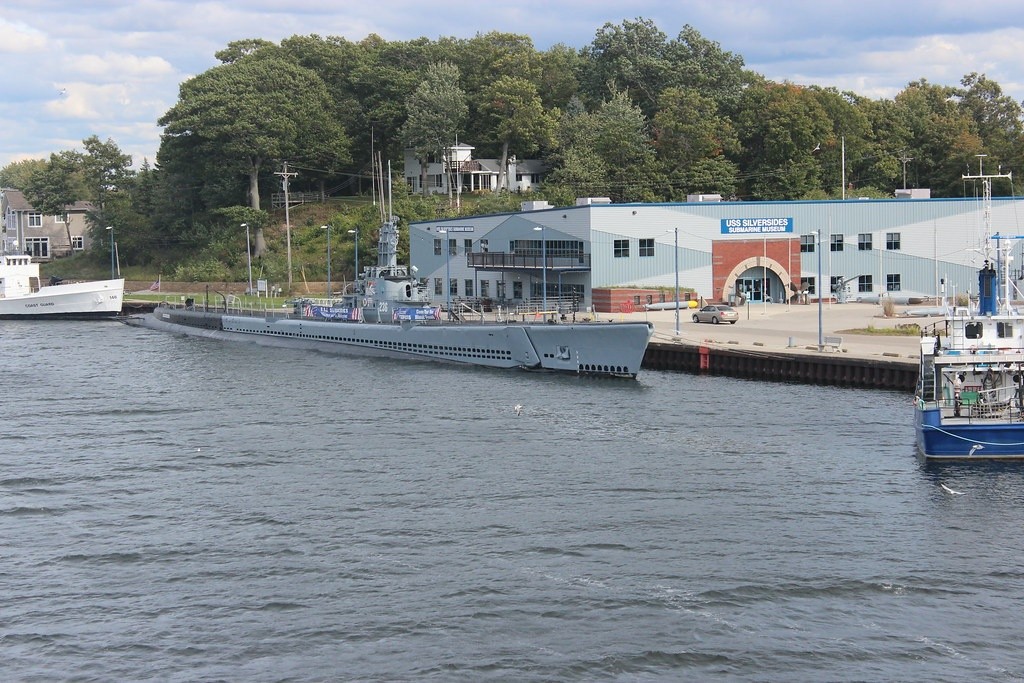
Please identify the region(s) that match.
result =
[126,215,655,379]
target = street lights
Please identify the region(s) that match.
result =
[808,229,826,352]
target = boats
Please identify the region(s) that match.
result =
[912,153,1024,466]
[0,252,125,320]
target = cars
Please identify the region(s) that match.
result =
[691,304,739,324]
